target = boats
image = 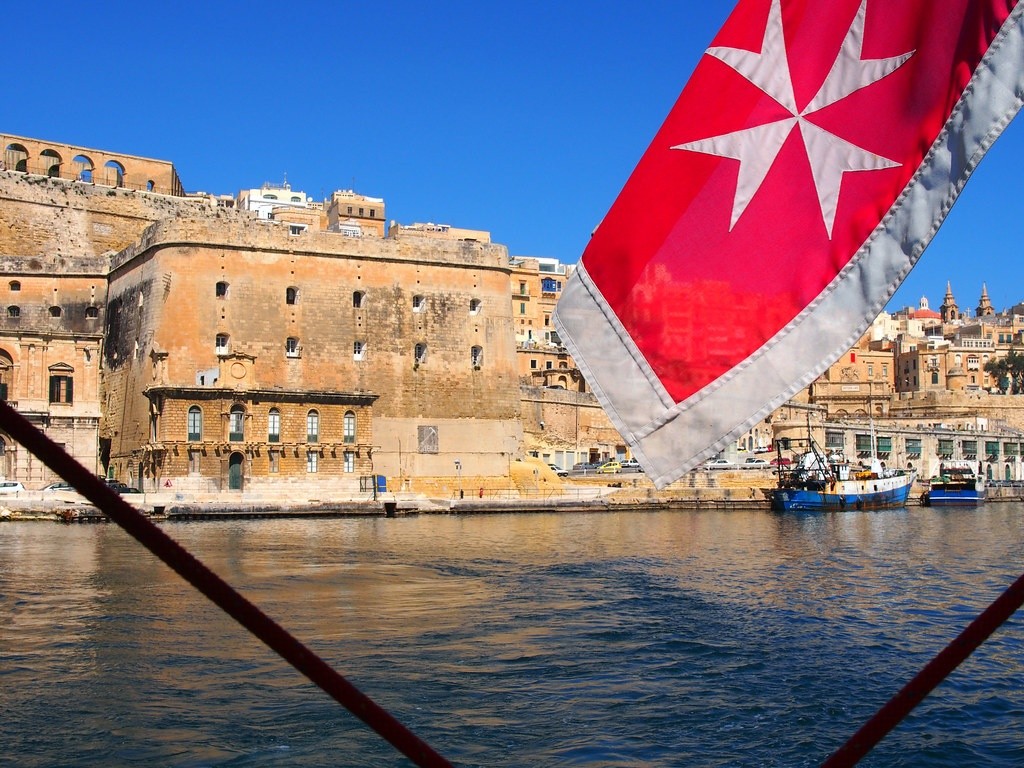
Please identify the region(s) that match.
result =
[760,408,919,511]
[919,460,987,507]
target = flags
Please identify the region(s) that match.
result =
[551,0,1024,491]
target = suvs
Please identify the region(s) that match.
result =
[548,464,568,477]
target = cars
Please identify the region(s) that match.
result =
[740,457,771,469]
[573,458,643,474]
[702,457,736,472]
[753,444,772,454]
[0,481,28,495]
[770,458,791,465]
[39,474,139,493]
